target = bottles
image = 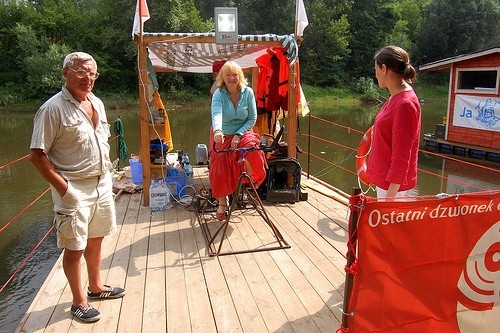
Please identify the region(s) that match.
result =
[183,162,193,186]
[182,153,189,162]
[149,180,171,211]
[165,169,186,197]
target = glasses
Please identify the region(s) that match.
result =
[67,68,100,79]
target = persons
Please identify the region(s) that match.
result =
[30,52,127,322]
[366,46,423,201]
[208,61,267,221]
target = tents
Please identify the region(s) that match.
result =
[132,33,303,206]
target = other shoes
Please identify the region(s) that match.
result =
[86,282,127,302]
[70,298,102,323]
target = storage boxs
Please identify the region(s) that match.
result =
[129,158,142,183]
[150,169,186,201]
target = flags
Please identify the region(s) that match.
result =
[131,0,151,40]
[296,0,309,36]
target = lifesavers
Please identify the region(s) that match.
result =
[355,125,375,186]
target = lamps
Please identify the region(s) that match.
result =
[214,7,238,45]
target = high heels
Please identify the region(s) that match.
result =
[216,204,227,220]
